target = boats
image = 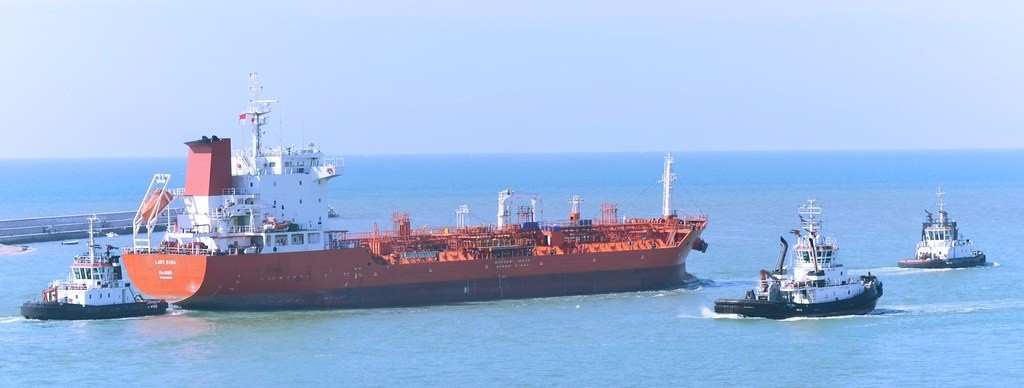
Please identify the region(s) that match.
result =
[121,72,709,310]
[713,197,884,321]
[19,213,169,320]
[897,187,987,269]
